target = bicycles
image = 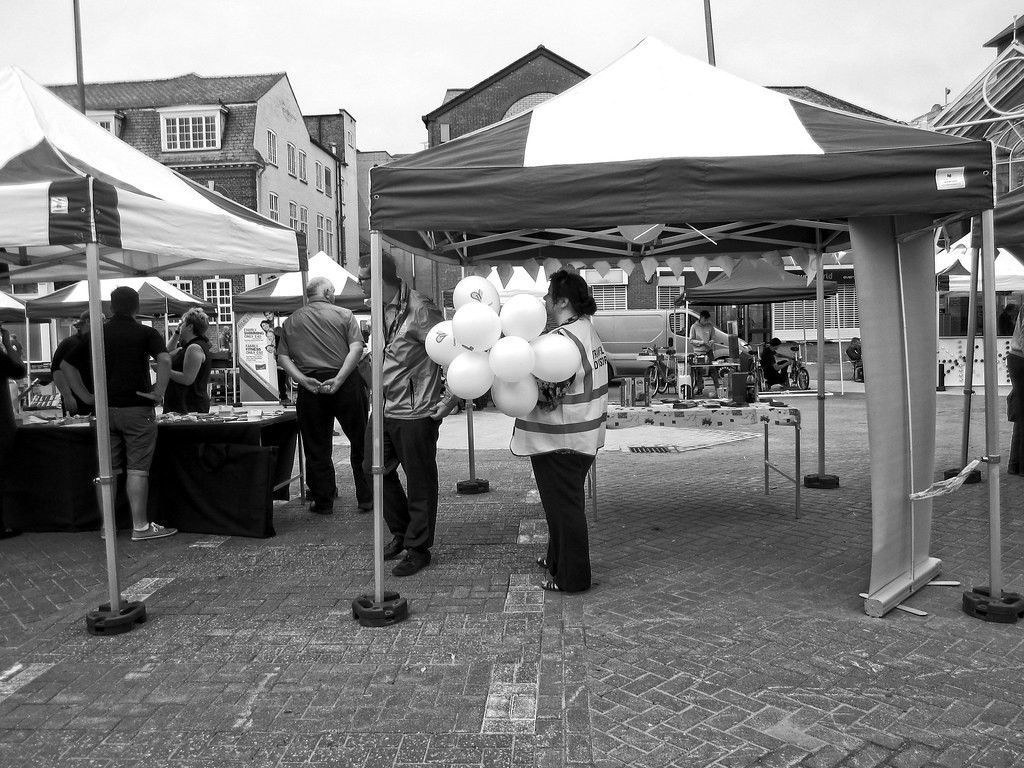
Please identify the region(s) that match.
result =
[785,340,816,391]
[640,344,678,400]
[744,341,771,391]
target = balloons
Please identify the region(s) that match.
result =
[426,276,583,417]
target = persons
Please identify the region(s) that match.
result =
[220,326,233,351]
[51,286,213,540]
[259,311,277,356]
[509,269,607,591]
[0,325,26,541]
[999,302,1024,476]
[274,327,291,405]
[356,252,460,576]
[762,338,794,392]
[278,278,374,514]
[689,311,719,395]
[846,337,861,366]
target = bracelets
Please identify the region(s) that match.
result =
[175,330,180,335]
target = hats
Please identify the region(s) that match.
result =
[74,310,106,328]
[770,338,782,346]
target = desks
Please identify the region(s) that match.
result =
[208,368,240,405]
[17,406,305,539]
[588,399,801,521]
[691,363,740,397]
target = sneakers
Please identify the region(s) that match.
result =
[132,522,178,540]
[100,523,116,539]
[384,535,405,559]
[392,549,431,576]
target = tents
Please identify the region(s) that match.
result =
[937,186,1024,469]
[674,257,844,400]
[232,250,371,406]
[934,231,1024,389]
[0,66,309,611]
[369,35,1001,601]
[0,275,220,407]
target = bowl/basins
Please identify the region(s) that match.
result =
[712,361,719,364]
[717,360,724,364]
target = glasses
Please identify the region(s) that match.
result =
[358,276,372,286]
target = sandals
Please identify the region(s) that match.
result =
[537,556,548,566]
[541,579,560,591]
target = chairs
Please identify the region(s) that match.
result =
[845,349,864,383]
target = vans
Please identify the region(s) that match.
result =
[590,307,753,382]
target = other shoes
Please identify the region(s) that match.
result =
[694,390,703,396]
[310,500,334,514]
[358,500,374,510]
